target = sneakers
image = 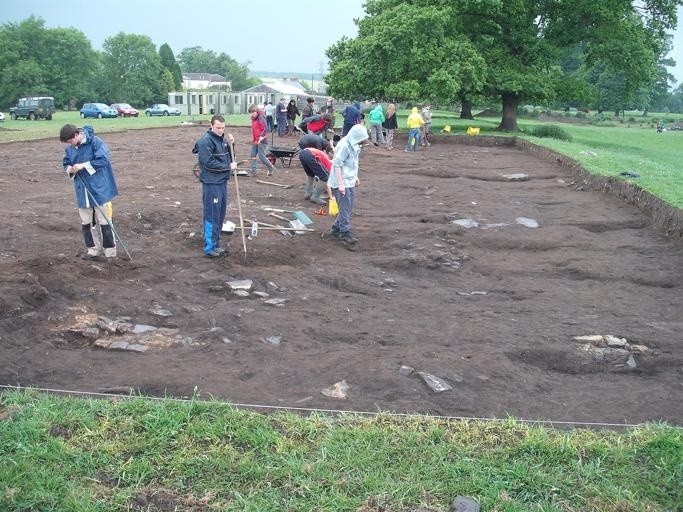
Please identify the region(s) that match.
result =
[208,247,230,257]
[331,226,358,244]
[81,253,98,260]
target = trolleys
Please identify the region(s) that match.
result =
[191,157,259,179]
[264,142,302,169]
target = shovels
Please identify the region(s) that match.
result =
[256,180,295,189]
[268,211,308,235]
[243,218,293,237]
[264,207,313,225]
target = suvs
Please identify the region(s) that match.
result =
[8,95,55,120]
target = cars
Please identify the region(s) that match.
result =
[144,103,182,116]
[0,111,5,121]
[110,102,139,117]
[79,102,117,118]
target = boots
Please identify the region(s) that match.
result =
[266,161,276,175]
[311,180,327,205]
[305,176,315,199]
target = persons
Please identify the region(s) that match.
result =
[192,115,238,260]
[298,146,333,206]
[58,123,120,259]
[247,96,433,175]
[325,122,370,246]
